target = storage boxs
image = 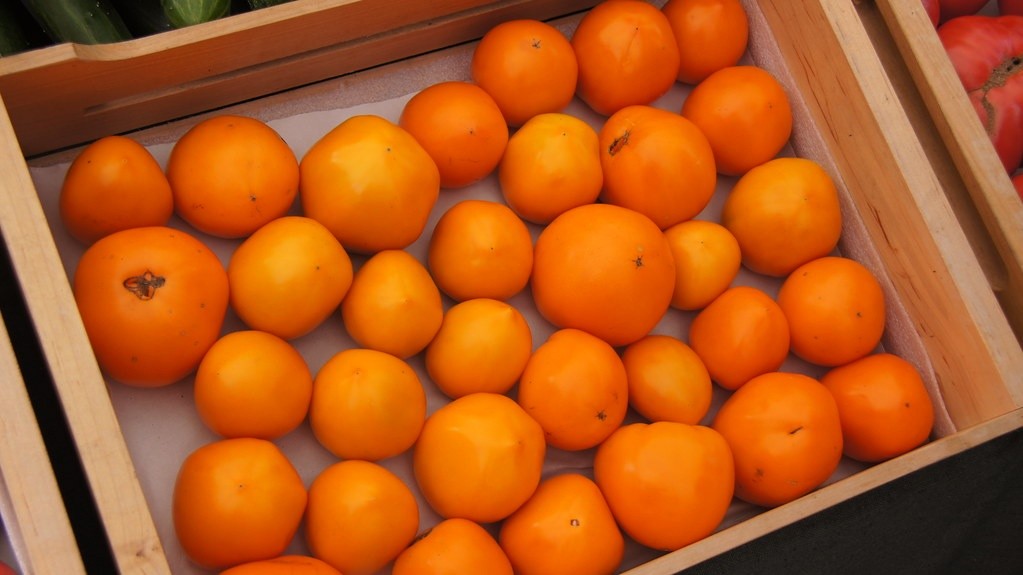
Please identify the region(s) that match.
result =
[1,0,1023,575]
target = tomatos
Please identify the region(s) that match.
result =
[58,0,1023,575]
